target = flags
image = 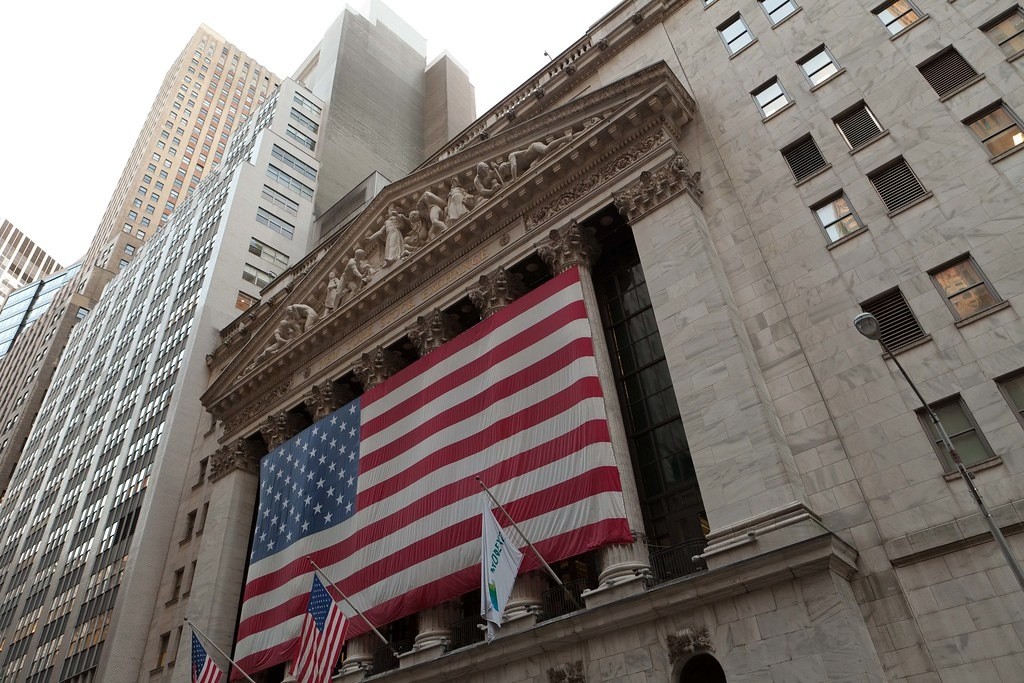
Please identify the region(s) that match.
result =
[190,629,225,683]
[225,261,637,681]
[480,494,525,629]
[287,571,351,683]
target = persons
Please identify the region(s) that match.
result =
[474,162,501,204]
[364,204,407,263]
[415,190,447,242]
[443,174,473,227]
[259,303,318,358]
[318,248,370,320]
[499,117,594,183]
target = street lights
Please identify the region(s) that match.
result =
[853,310,1024,590]
